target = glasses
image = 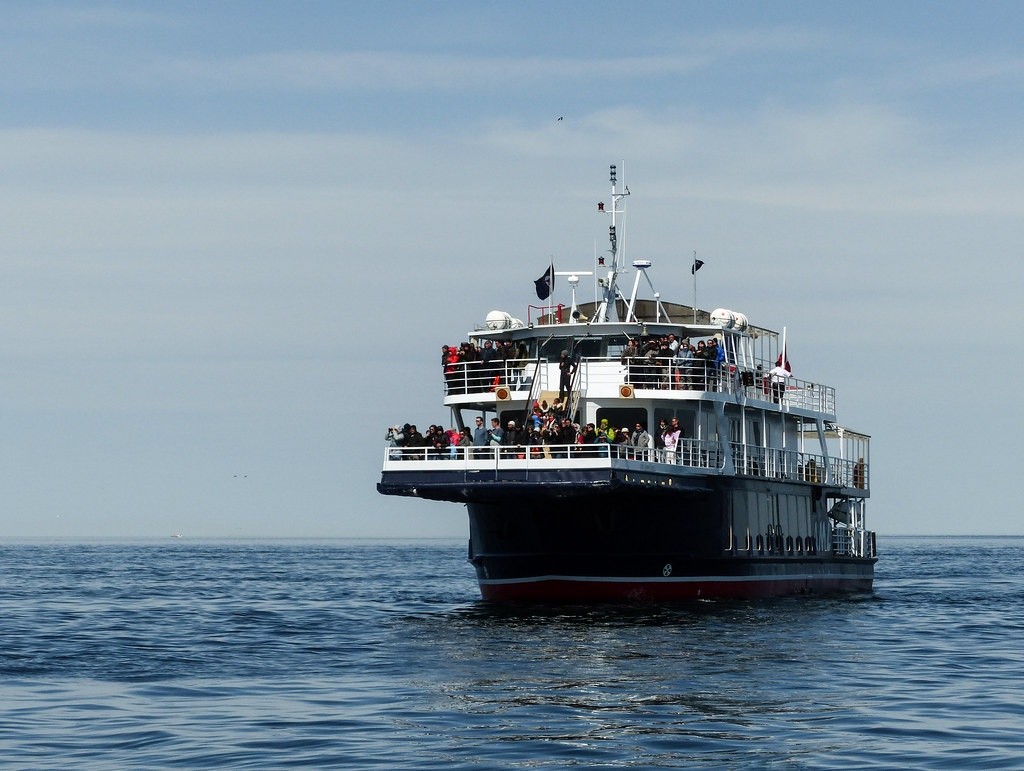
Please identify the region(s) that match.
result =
[636,426,641,427]
[430,429,435,431]
[708,343,712,344]
[587,427,592,428]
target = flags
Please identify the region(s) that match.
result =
[534,264,555,302]
[775,343,793,377]
[692,259,705,275]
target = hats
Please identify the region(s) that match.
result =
[561,350,569,356]
[621,428,629,432]
[442,345,448,349]
[601,419,609,424]
[508,421,516,426]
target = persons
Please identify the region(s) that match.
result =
[385,332,863,488]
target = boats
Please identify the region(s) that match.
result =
[374,157,879,605]
[833,499,860,523]
